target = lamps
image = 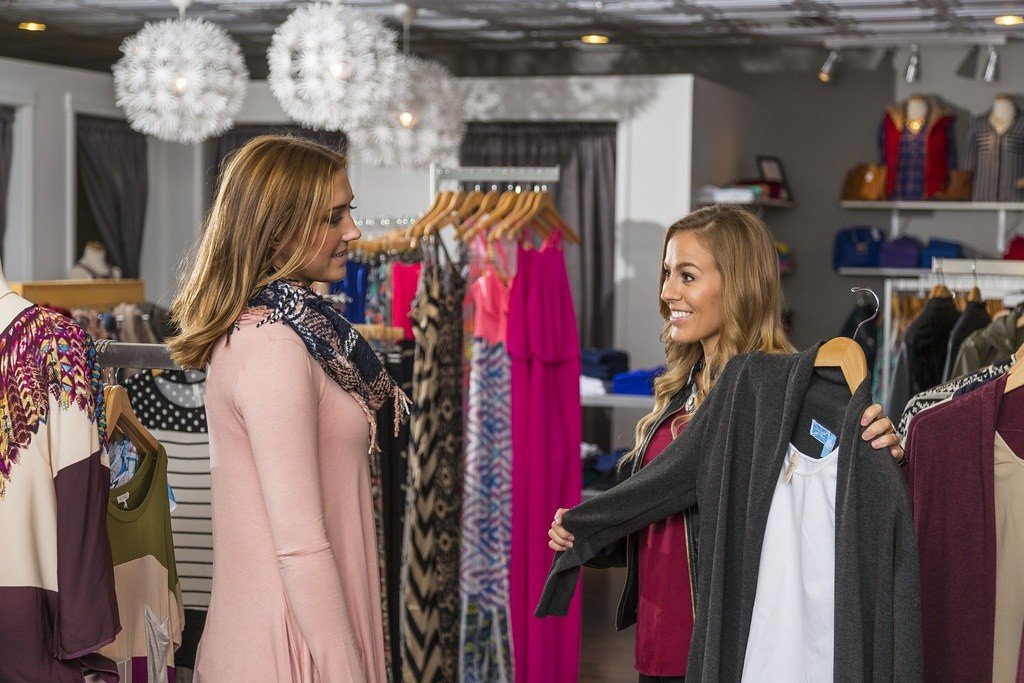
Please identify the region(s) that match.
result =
[981,44,1001,83]
[819,46,842,84]
[903,45,923,84]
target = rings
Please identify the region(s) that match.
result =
[886,416,898,434]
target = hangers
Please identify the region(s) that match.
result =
[813,257,1024,392]
[91,335,161,454]
[345,163,583,266]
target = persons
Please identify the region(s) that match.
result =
[546,203,906,683]
[877,95,959,202]
[958,90,1024,202]
[166,137,414,682]
[0,260,122,683]
[72,242,121,282]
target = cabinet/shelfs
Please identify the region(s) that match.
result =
[838,202,1024,275]
[577,394,658,505]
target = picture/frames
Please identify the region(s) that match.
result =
[755,154,791,200]
